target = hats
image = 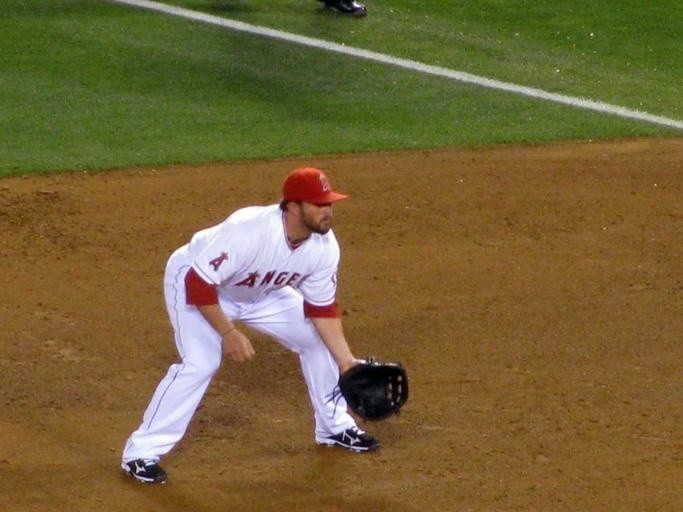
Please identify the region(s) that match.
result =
[282,168,349,205]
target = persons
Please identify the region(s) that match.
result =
[118,166,380,484]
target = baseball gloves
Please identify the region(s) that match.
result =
[339,362,409,422]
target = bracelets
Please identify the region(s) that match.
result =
[217,325,239,338]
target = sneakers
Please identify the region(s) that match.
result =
[315,425,380,453]
[121,457,167,485]
